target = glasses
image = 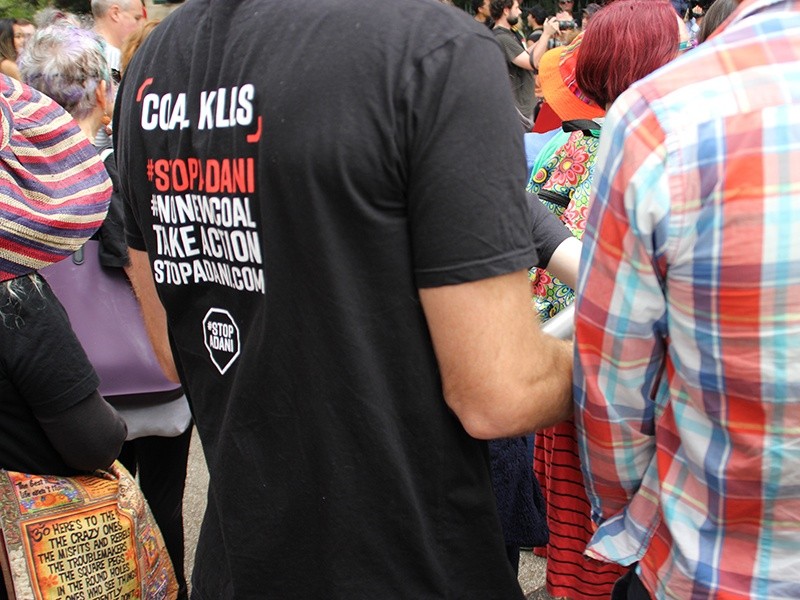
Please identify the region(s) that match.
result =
[560,0,572,4]
[111,68,121,86]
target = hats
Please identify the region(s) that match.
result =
[0,72,113,283]
[538,29,606,121]
[582,3,602,18]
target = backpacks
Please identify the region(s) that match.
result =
[524,119,602,326]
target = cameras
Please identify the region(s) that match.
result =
[548,15,574,31]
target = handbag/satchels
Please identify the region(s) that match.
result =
[37,148,184,407]
[0,459,180,600]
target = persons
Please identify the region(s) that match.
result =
[0,0,799,600]
